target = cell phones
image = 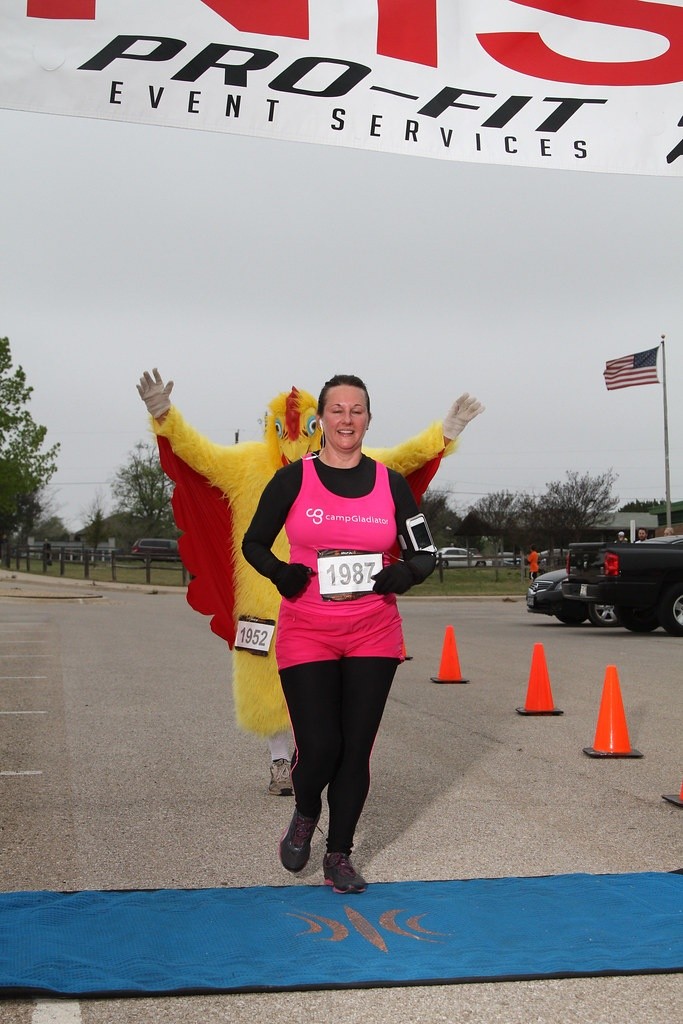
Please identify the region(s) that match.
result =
[406,513,438,552]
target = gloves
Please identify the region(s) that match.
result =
[136,368,174,418]
[442,392,486,440]
[271,562,313,599]
[371,563,411,594]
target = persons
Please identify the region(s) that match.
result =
[136,367,486,796]
[635,528,648,543]
[527,545,538,582]
[243,375,436,895]
[613,531,627,543]
[42,539,51,565]
[664,527,674,536]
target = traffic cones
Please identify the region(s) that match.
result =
[401,641,414,661]
[660,774,683,810]
[515,643,564,717]
[428,624,470,683]
[582,665,645,759]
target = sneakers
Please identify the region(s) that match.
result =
[268,758,294,796]
[280,806,322,873]
[322,852,366,894]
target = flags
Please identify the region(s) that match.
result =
[603,348,663,390]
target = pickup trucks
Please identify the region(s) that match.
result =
[562,541,683,638]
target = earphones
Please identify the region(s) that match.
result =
[319,419,323,430]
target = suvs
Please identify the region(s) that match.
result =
[129,537,182,564]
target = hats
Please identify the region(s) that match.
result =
[617,532,624,536]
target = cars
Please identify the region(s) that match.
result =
[526,535,683,627]
[497,551,522,567]
[435,547,493,569]
